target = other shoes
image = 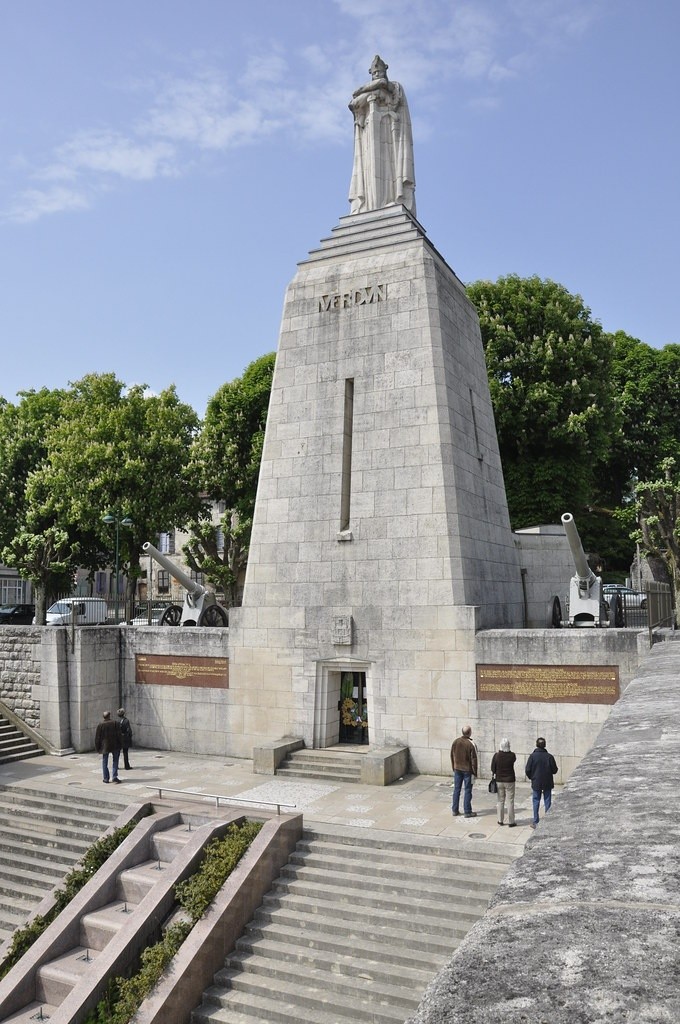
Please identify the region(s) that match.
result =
[497,821,503,826]
[464,812,477,818]
[509,824,517,827]
[112,778,121,783]
[530,823,538,829]
[130,767,133,770]
[452,811,459,816]
[103,780,109,783]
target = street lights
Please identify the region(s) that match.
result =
[102,509,133,626]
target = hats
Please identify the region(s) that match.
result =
[117,708,124,715]
[500,738,510,753]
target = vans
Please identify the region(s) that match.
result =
[32,596,108,626]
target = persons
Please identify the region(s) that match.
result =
[115,708,133,770]
[524,737,558,830]
[95,711,121,784]
[488,738,517,827]
[449,726,478,818]
[347,54,417,217]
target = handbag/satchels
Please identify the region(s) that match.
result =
[488,774,497,793]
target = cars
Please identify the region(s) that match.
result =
[0,603,35,625]
[134,600,173,618]
[118,607,179,626]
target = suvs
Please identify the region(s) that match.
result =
[602,584,648,612]
